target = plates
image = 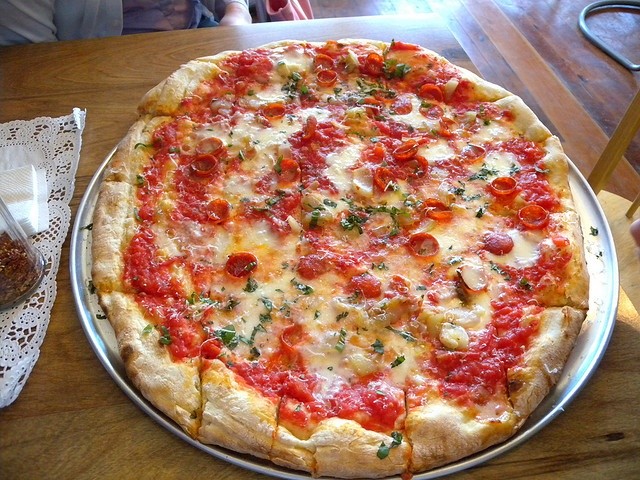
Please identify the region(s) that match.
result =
[68,137,620,480]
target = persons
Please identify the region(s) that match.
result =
[1,0,253,46]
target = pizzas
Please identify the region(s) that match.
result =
[90,37,590,479]
